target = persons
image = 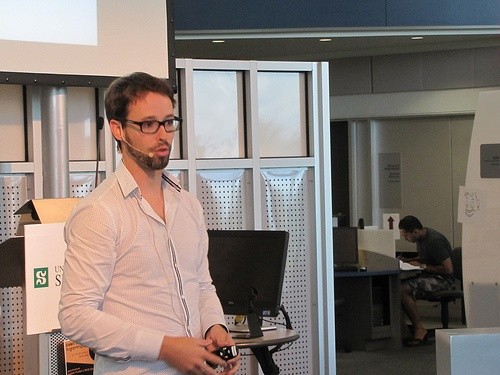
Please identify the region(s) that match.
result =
[399,216,454,345]
[58,72,241,375]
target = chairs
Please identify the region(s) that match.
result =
[417,247,463,330]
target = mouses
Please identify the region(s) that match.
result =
[359,266,367,271]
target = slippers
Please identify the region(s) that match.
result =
[408,331,428,346]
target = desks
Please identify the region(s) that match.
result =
[227,323,300,375]
[334,232,421,351]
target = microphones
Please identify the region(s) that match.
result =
[121,134,153,158]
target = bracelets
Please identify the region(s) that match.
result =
[422,264,426,271]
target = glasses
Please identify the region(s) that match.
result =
[113,116,183,134]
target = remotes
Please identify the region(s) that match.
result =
[206,345,238,368]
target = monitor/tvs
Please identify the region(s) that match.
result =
[333,226,357,265]
[207,230,289,316]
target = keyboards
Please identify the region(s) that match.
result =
[334,266,358,272]
